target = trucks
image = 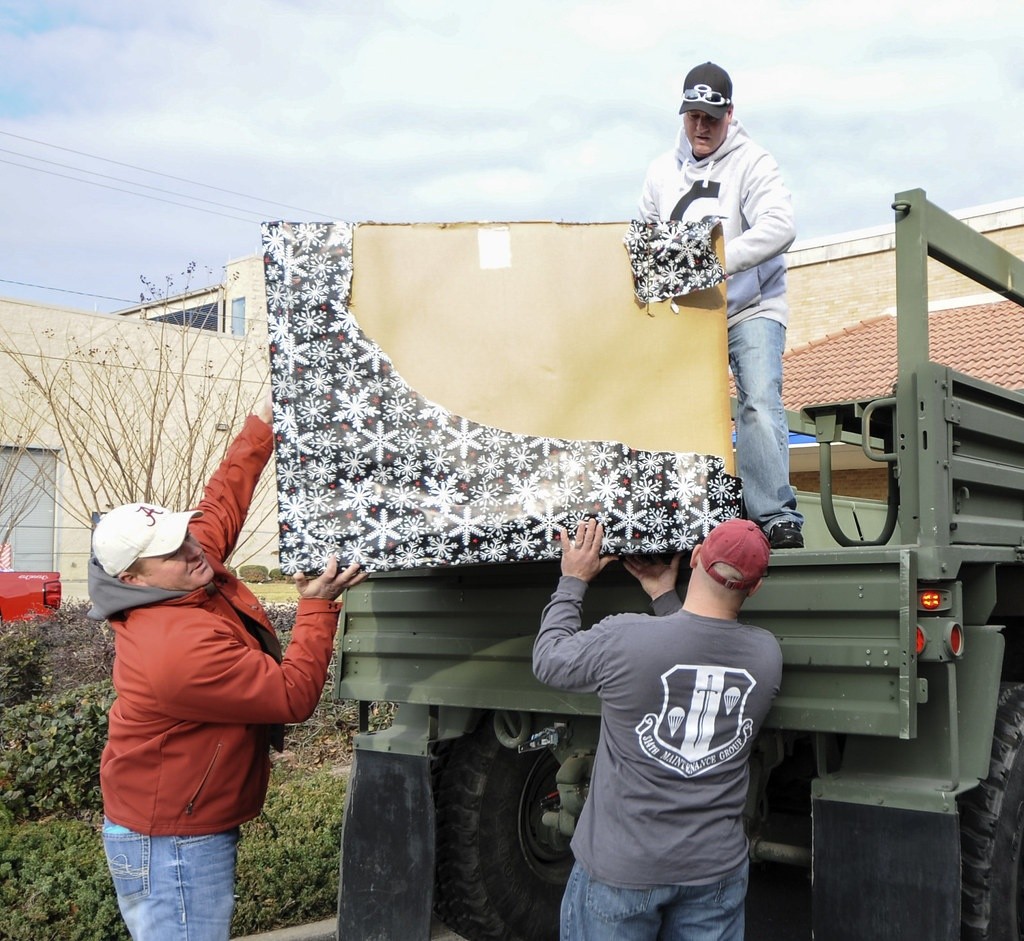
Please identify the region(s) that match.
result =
[322,185,1024,941]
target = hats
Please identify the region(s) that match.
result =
[91,503,205,577]
[700,518,770,588]
[678,62,732,119]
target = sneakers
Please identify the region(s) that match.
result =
[769,522,806,548]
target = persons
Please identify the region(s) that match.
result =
[531,515,786,941]
[636,64,806,554]
[86,375,371,941]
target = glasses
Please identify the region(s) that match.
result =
[683,88,729,106]
